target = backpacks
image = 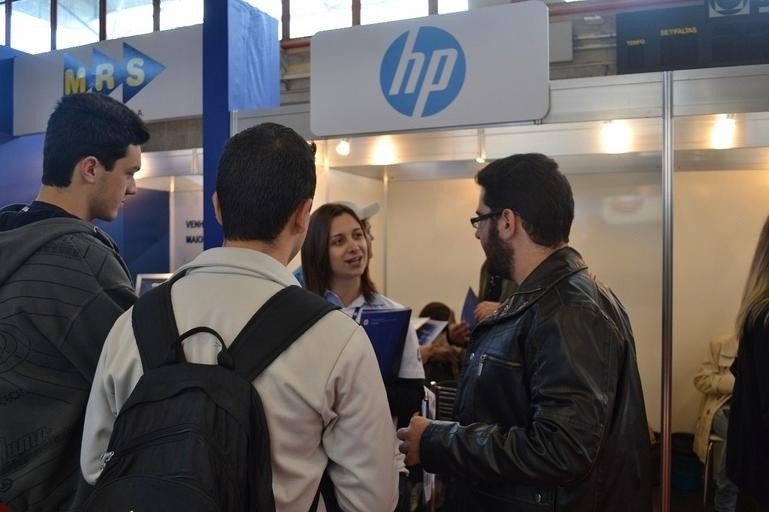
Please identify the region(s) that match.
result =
[80,267,342,512]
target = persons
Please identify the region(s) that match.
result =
[0,93,150,512]
[80,122,400,512]
[397,153,650,512]
[726,217,769,512]
[693,334,739,512]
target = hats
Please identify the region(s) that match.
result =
[324,199,383,221]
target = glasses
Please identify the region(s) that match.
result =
[470,209,516,230]
